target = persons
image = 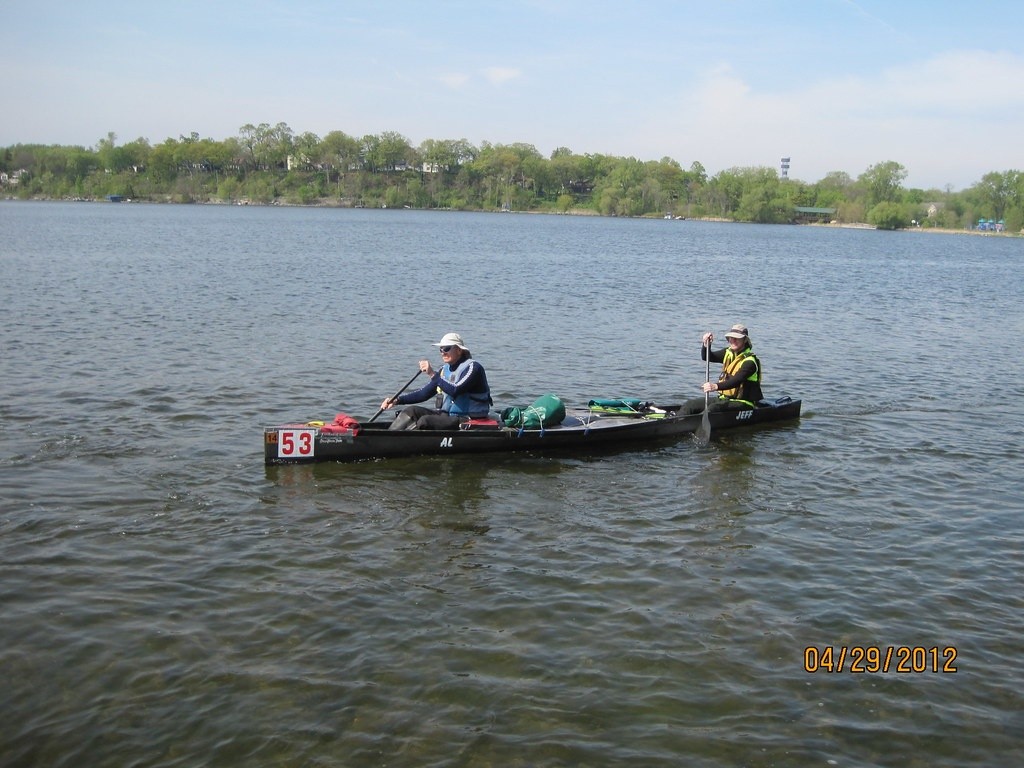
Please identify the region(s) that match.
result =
[380,333,493,430]
[678,324,764,415]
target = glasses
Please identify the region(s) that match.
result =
[439,346,455,352]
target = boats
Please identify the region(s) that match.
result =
[263,394,802,467]
[105,195,123,202]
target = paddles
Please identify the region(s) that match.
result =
[693,335,713,445]
[365,370,427,422]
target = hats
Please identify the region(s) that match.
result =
[431,333,472,359]
[724,324,752,350]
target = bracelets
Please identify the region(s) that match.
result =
[431,372,436,378]
